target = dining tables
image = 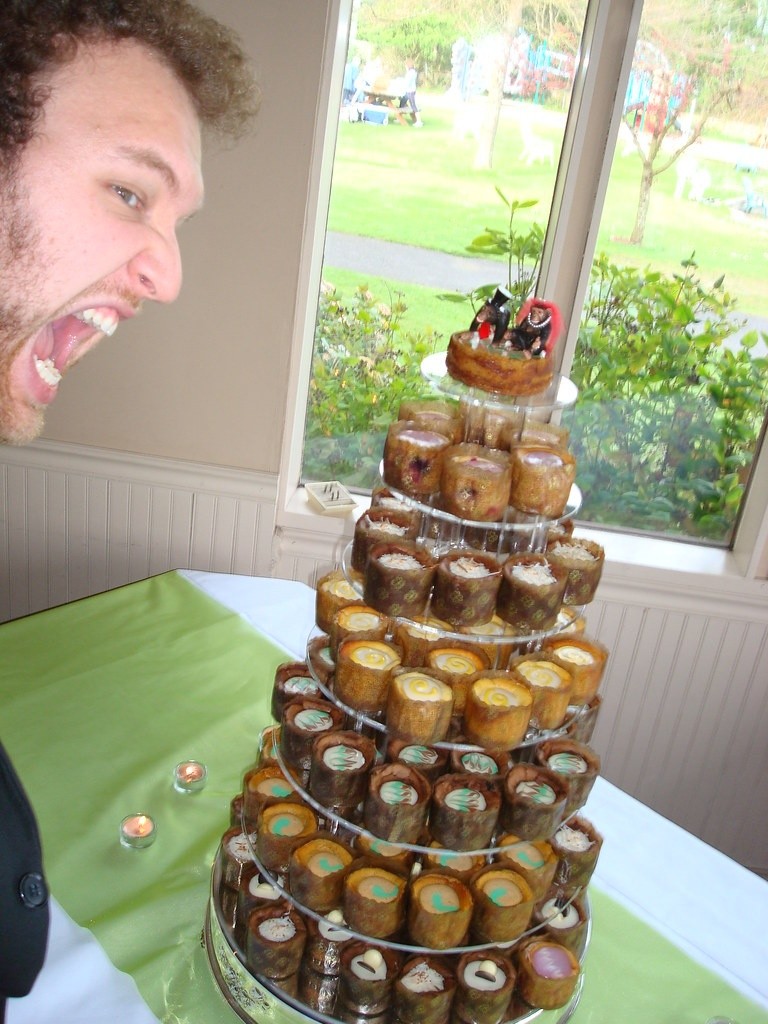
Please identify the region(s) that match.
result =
[0,570,766,1024]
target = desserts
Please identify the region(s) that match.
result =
[215,324,612,1023]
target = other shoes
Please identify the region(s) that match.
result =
[414,121,424,127]
[393,119,399,123]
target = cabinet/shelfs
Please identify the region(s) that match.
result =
[204,356,606,1023]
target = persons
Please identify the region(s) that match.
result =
[394,60,422,127]
[343,55,361,99]
[1,1,251,1024]
[350,55,384,120]
